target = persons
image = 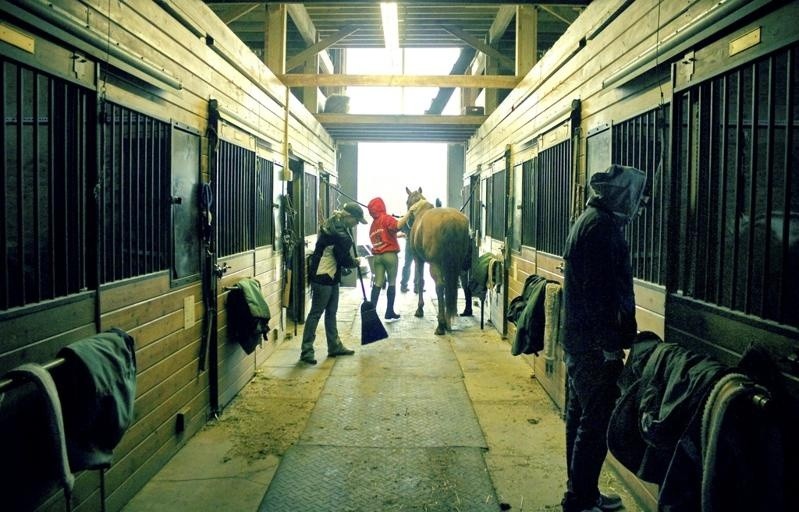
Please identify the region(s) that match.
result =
[300,202,367,364]
[368,197,418,319]
[458,236,474,316]
[401,213,426,293]
[561,164,648,511]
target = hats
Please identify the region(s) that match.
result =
[343,202,368,225]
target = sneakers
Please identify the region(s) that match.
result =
[596,492,623,510]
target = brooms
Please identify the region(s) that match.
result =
[350,228,388,346]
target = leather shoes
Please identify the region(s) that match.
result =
[328,348,356,357]
[303,357,317,364]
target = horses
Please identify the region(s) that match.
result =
[406,186,470,335]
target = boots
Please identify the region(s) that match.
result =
[371,285,380,307]
[384,285,400,323]
[461,287,473,317]
